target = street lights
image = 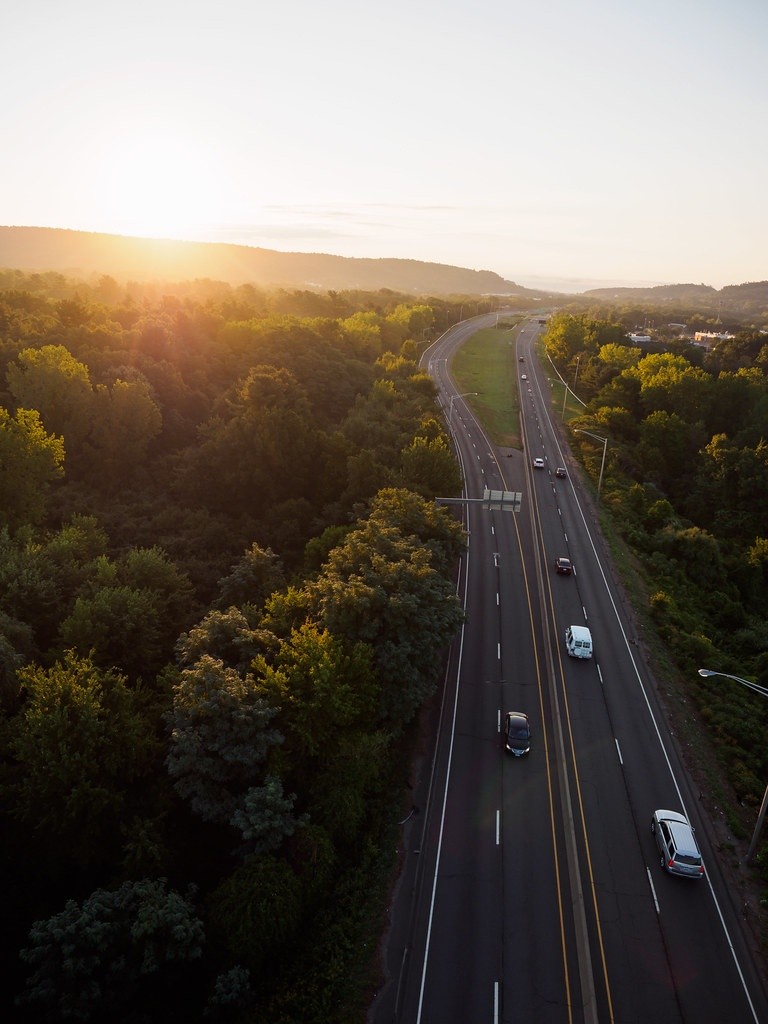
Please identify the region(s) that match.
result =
[698,669,768,863]
[448,393,478,437]
[549,378,568,421]
[428,359,448,377]
[575,430,607,503]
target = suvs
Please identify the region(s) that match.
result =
[651,809,704,879]
[533,458,544,469]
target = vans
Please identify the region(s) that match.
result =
[565,625,592,660]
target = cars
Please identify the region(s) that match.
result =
[503,712,531,760]
[555,557,572,575]
[522,375,526,379]
[519,357,525,361]
[556,468,567,478]
